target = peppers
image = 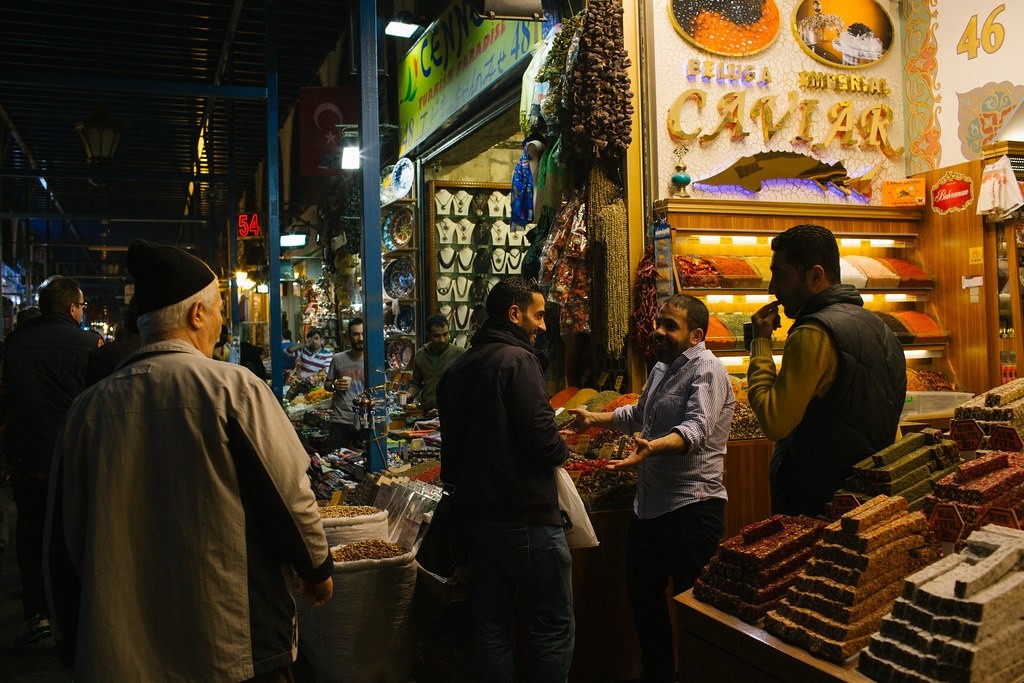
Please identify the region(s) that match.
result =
[626,244,663,361]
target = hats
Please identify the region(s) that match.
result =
[215,324,228,347]
[125,238,218,317]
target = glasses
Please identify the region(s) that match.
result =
[73,301,88,309]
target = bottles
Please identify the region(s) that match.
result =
[90,320,103,332]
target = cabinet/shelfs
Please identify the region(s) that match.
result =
[654,200,975,560]
[669,576,875,683]
[379,159,419,376]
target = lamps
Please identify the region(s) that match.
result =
[340,139,360,170]
[231,265,363,311]
[385,10,426,40]
[279,224,321,248]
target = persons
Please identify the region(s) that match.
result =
[212,324,265,382]
[0,275,143,646]
[558,295,737,683]
[42,239,334,683]
[281,327,333,386]
[398,314,466,417]
[436,276,575,683]
[324,317,389,455]
[747,225,907,519]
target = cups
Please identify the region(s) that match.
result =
[343,376,352,390]
[398,393,408,406]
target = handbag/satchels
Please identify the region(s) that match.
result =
[555,464,601,550]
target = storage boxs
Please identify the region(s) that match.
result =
[674,178,974,442]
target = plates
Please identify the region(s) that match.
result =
[380,210,396,252]
[387,339,414,371]
[391,158,414,198]
[384,257,416,299]
[380,165,394,203]
[384,306,414,334]
[390,206,412,247]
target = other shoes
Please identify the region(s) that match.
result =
[13,613,54,650]
